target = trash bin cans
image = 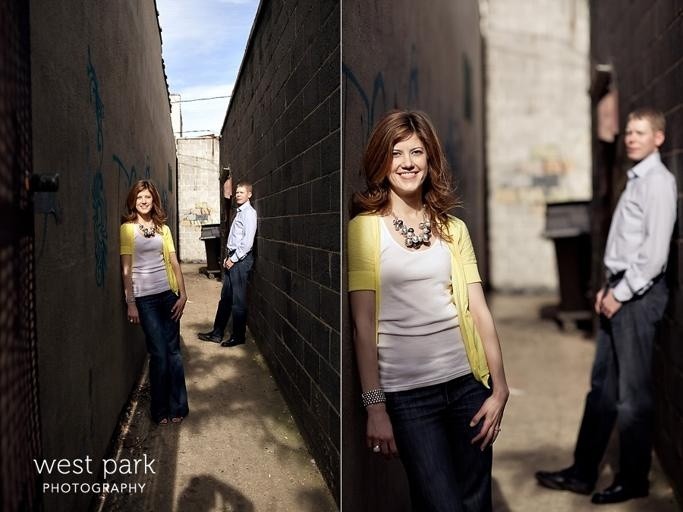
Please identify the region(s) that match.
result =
[541,200,596,333]
[199,224,221,279]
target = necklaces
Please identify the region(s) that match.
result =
[390,212,431,249]
[136,220,155,238]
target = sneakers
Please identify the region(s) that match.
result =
[221,336,246,347]
[198,330,222,343]
[534,465,595,496]
[591,479,650,505]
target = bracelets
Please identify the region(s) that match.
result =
[361,388,387,407]
[124,296,135,304]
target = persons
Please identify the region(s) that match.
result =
[534,106,678,504]
[120,179,188,423]
[197,181,257,346]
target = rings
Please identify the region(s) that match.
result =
[130,320,133,322]
[495,428,501,431]
[371,444,380,452]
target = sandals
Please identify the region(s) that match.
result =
[158,414,185,424]
